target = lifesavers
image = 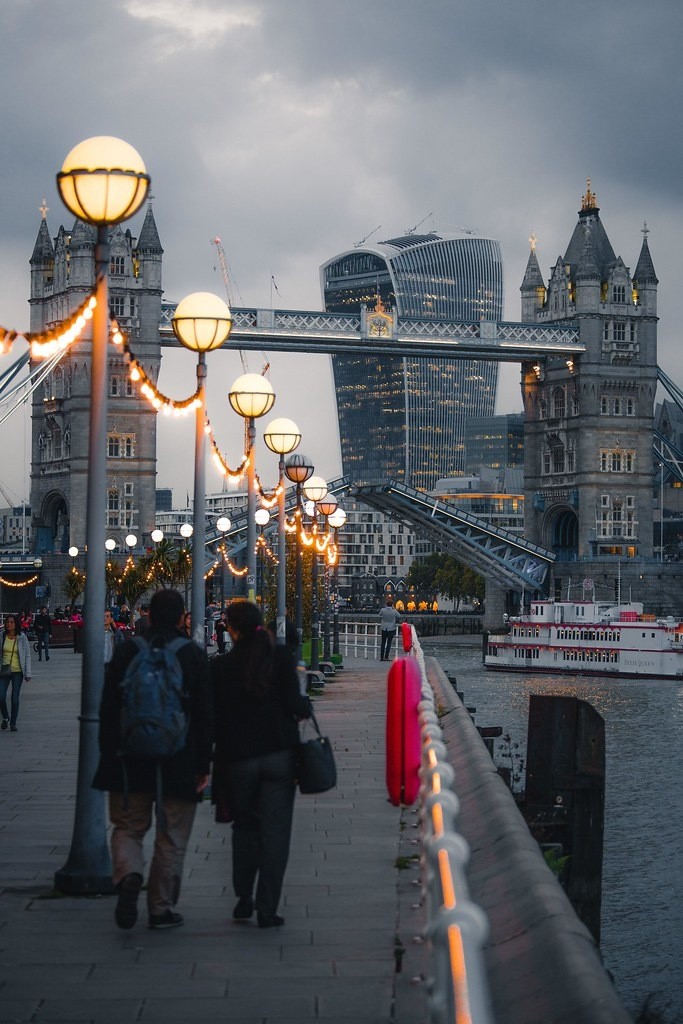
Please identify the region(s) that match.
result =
[384,658,422,810]
[402,622,412,652]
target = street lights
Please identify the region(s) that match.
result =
[316,492,339,677]
[53,133,150,897]
[285,452,315,697]
[169,291,233,659]
[151,529,164,593]
[179,524,193,613]
[33,558,43,608]
[217,517,232,613]
[125,534,138,556]
[302,475,328,688]
[263,417,302,645]
[255,508,271,604]
[328,508,346,669]
[228,373,276,604]
[105,539,116,607]
[68,546,79,603]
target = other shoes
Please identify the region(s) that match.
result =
[1,717,10,729]
[380,658,385,661]
[207,643,213,647]
[11,726,17,731]
[258,914,284,927]
[46,656,49,661]
[385,658,392,661]
[39,658,42,661]
[232,897,254,919]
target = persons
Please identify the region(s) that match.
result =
[185,613,191,638]
[135,604,149,635]
[121,605,129,623]
[0,615,31,731]
[54,608,63,619]
[379,600,401,661]
[267,606,299,656]
[210,603,312,925]
[70,610,80,621]
[215,611,227,654]
[92,589,211,929]
[103,610,125,694]
[206,602,220,647]
[65,605,70,619]
[33,607,52,662]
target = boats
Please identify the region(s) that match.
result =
[482,558,683,680]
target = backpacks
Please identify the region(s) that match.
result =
[119,634,194,758]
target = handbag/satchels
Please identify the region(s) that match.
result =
[0,664,11,677]
[296,700,336,793]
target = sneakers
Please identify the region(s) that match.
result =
[149,909,185,929]
[115,875,142,929]
